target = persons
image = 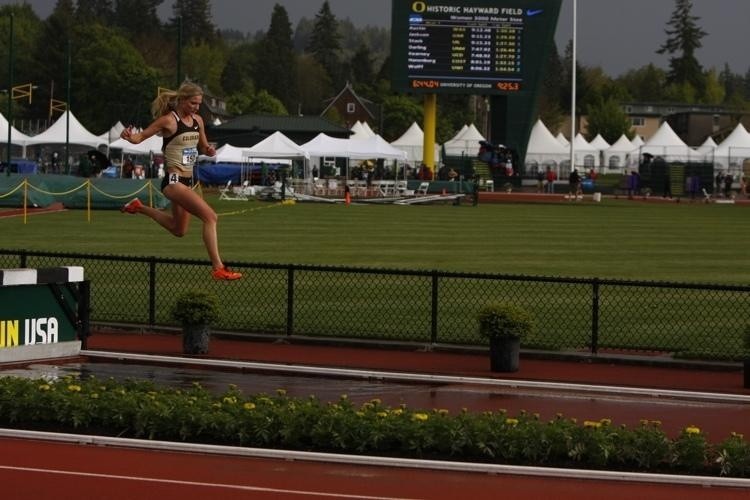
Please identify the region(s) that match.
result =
[119,81,243,281]
[2,146,749,209]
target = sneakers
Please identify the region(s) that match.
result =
[211,267,242,280]
[120,197,143,214]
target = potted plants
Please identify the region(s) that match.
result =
[170,287,222,354]
[474,301,535,373]
